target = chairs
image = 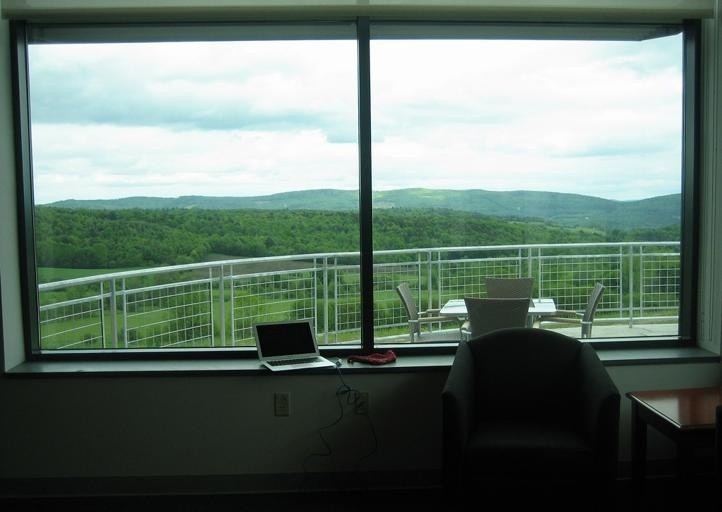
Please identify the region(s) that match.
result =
[397,280,464,342]
[535,281,607,338]
[459,296,531,340]
[484,275,537,297]
[431,328,624,511]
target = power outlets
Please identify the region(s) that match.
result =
[352,392,372,415]
[274,391,291,416]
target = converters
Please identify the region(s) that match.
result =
[335,358,343,366]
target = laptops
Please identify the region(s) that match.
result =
[254,319,336,372]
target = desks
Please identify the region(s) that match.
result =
[623,382,722,512]
[437,292,557,318]
